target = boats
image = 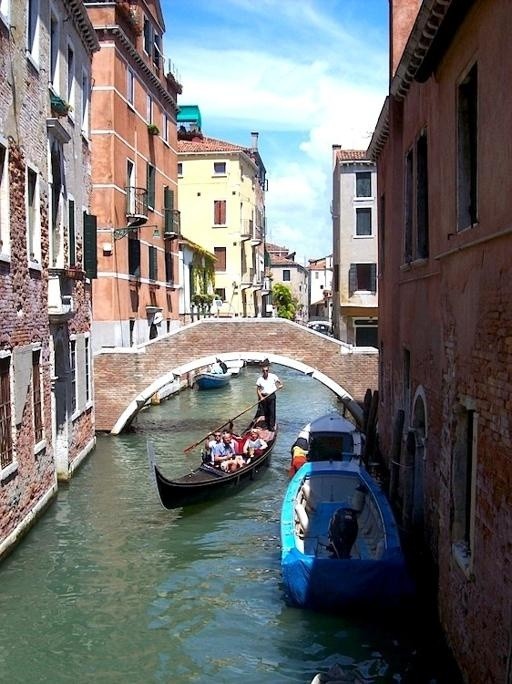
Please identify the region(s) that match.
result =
[306,320,332,336]
[152,412,279,510]
[279,459,405,611]
[289,406,368,479]
[194,371,232,392]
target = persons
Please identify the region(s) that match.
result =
[205,418,275,472]
[253,366,283,432]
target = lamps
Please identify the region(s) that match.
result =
[114,225,160,241]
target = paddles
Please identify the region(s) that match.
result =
[182,388,281,452]
